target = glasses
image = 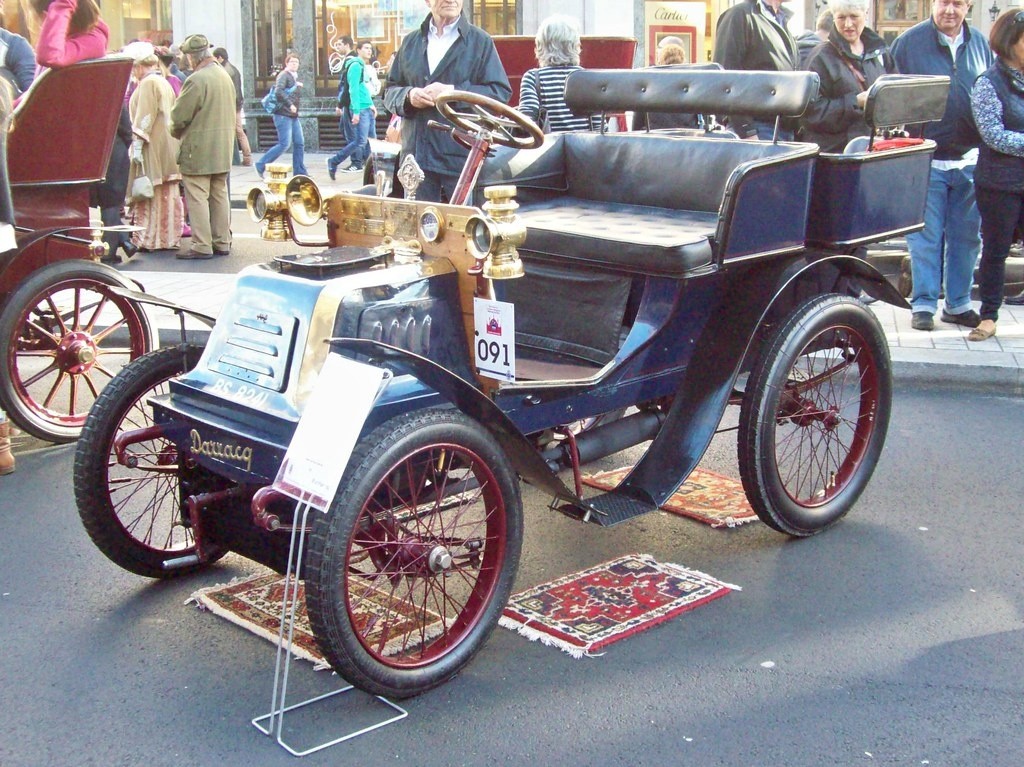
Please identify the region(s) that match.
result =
[1011,11,1024,24]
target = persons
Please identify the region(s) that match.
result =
[338,36,364,171]
[327,40,378,180]
[0,0,252,258]
[383,0,513,205]
[498,17,609,137]
[390,51,396,63]
[256,53,312,178]
[630,0,1024,341]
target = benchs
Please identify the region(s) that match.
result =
[569,62,740,139]
[0,53,136,189]
[511,68,821,273]
[807,73,951,249]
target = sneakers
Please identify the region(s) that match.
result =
[340,163,363,172]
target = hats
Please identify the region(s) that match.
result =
[178,34,209,53]
[123,40,154,64]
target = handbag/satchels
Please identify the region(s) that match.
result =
[131,161,154,202]
[261,78,297,114]
[504,68,550,139]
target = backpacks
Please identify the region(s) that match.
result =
[338,60,364,106]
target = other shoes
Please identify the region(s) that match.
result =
[858,294,877,304]
[911,311,934,331]
[940,308,982,328]
[969,325,997,341]
[1008,239,1024,257]
[898,256,914,297]
[212,246,229,255]
[181,222,192,237]
[255,162,265,179]
[328,159,336,180]
[1003,289,1024,305]
[176,248,213,259]
[123,240,137,258]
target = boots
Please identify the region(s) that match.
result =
[0,421,16,475]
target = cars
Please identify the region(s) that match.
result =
[0,59,161,442]
[72,63,955,701]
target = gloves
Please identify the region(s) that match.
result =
[133,139,145,164]
